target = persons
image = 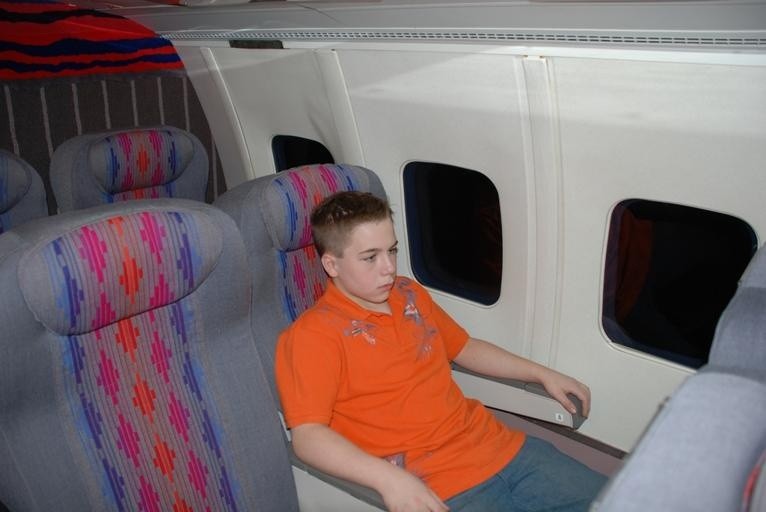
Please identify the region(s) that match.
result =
[275,191,611,512]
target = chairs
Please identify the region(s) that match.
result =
[590,235,766,511]
[213,162,589,512]
[0,197,382,512]
[0,148,47,228]
[48,125,210,210]
